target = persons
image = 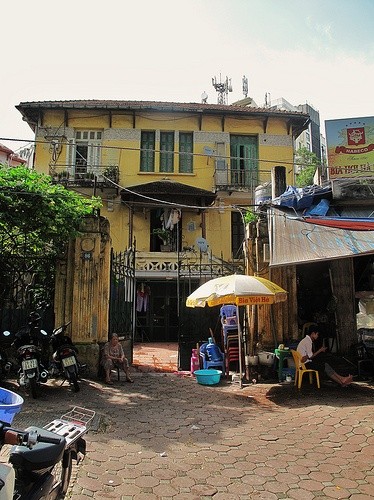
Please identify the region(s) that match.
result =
[101,333,134,385]
[295,324,353,388]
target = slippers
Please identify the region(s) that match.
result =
[105,381,114,385]
[126,378,134,382]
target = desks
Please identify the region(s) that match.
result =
[275,348,297,382]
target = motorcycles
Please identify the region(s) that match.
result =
[0,405,98,500]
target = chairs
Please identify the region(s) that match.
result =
[97,339,124,381]
[190,304,245,376]
[290,349,321,389]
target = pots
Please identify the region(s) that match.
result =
[259,351,275,367]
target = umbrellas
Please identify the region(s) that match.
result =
[186,273,288,388]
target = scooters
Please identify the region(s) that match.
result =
[2,311,50,399]
[40,320,88,393]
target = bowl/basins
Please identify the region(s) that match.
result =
[193,368,223,385]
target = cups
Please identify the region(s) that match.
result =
[286,374,292,382]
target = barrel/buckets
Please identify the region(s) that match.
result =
[253,183,272,211]
[0,386,24,427]
